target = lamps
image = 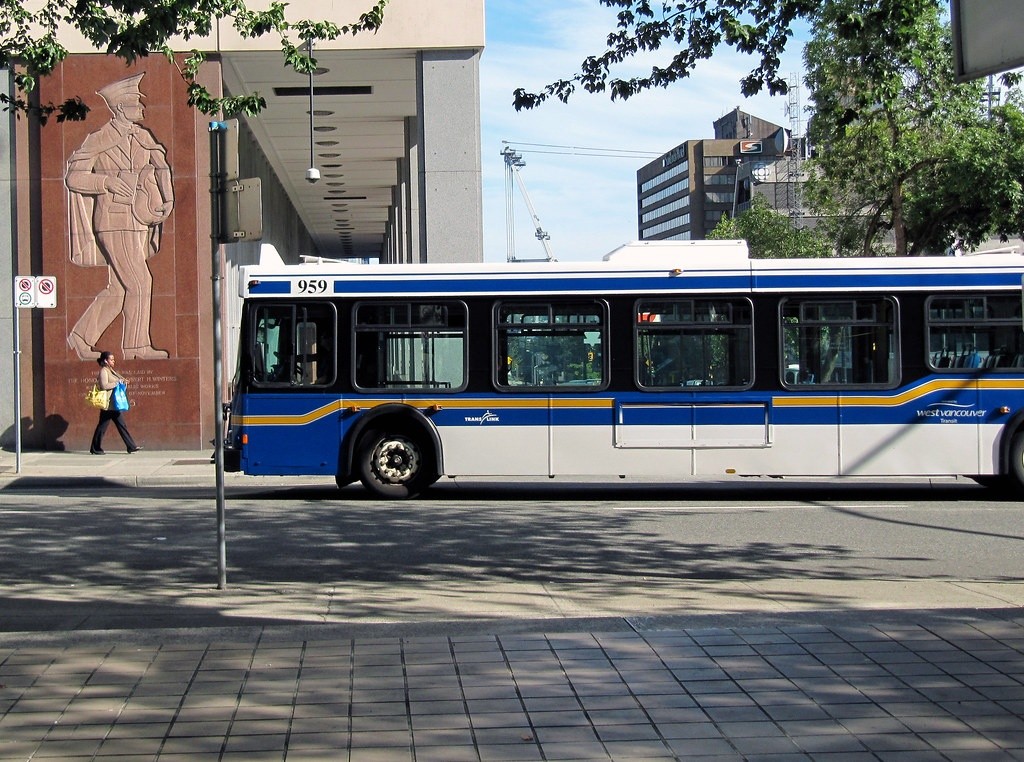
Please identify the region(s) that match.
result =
[305,35,322,183]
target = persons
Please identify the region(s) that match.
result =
[89,351,145,455]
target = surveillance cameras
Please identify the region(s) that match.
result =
[305,168,321,184]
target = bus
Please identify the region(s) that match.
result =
[211,236,1024,504]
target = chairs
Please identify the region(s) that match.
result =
[786,372,840,384]
[931,344,1024,368]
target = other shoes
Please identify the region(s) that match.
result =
[128,446,143,454]
[90,449,104,455]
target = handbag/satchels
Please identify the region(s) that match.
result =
[85,368,112,410]
[112,381,129,410]
